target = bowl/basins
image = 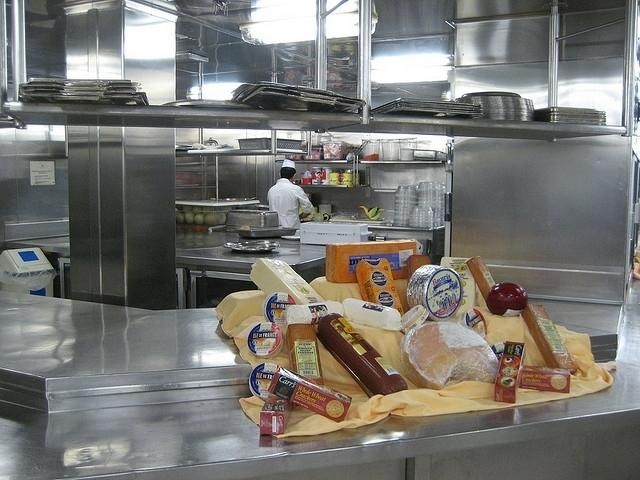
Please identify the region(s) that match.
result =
[393,182,444,228]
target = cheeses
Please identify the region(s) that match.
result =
[440,256,477,318]
[249,258,325,305]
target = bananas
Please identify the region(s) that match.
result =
[361,205,385,220]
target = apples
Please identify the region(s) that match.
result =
[485,282,529,315]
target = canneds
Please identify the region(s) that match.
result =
[301,167,353,187]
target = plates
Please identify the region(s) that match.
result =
[223,240,280,253]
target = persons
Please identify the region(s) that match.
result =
[267,157,314,236]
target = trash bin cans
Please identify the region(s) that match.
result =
[0,247,54,297]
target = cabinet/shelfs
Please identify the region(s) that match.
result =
[360,160,446,194]
[274,159,357,190]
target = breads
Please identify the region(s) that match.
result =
[398,322,499,390]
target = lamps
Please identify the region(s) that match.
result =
[238,10,377,45]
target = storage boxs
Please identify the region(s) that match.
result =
[300,221,369,246]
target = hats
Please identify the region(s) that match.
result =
[280,159,298,172]
